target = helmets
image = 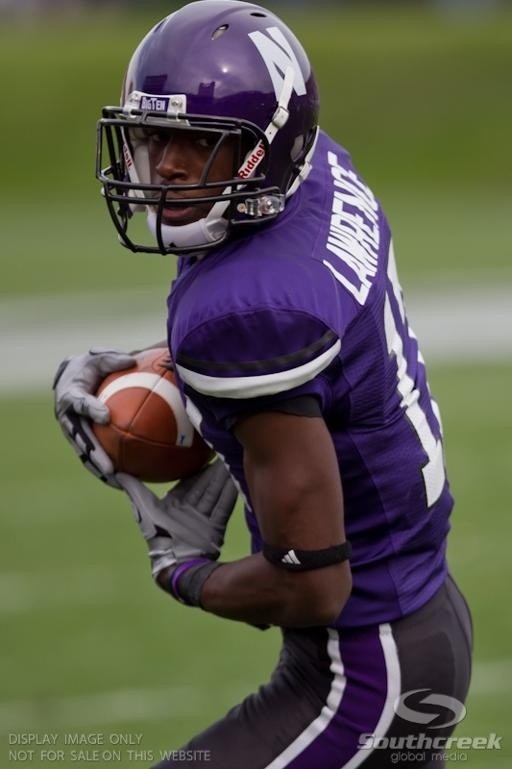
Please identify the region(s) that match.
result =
[95,1,320,255]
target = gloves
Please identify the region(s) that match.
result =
[51,349,137,480]
[114,460,238,608]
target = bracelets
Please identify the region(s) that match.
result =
[173,558,222,607]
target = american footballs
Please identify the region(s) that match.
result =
[90,346,215,485]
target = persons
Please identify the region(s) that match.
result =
[51,0,475,769]
